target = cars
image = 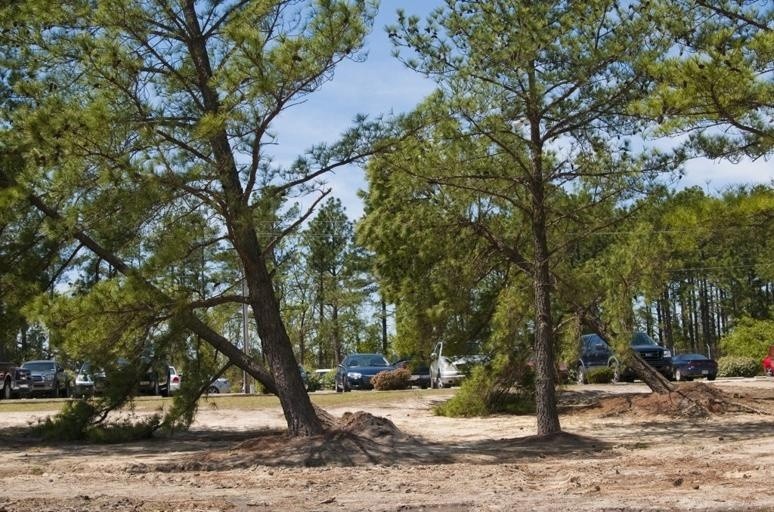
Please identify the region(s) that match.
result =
[577,332,718,383]
[334,353,396,393]
[428,339,490,388]
[0,360,233,395]
